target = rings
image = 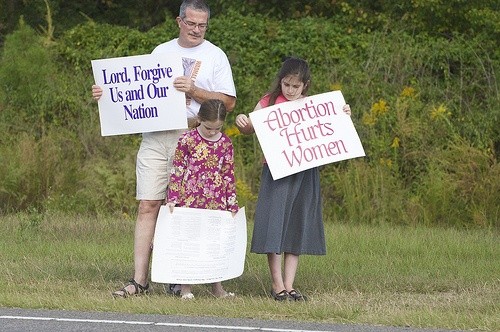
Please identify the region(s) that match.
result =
[183,84,184,87]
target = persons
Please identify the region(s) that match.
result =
[93,0,236,297]
[236,59,351,301]
[165,99,239,299]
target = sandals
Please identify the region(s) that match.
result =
[112,278,149,298]
[168,283,182,298]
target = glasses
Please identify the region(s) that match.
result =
[180,16,210,31]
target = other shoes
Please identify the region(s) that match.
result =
[288,289,307,301]
[271,289,295,302]
